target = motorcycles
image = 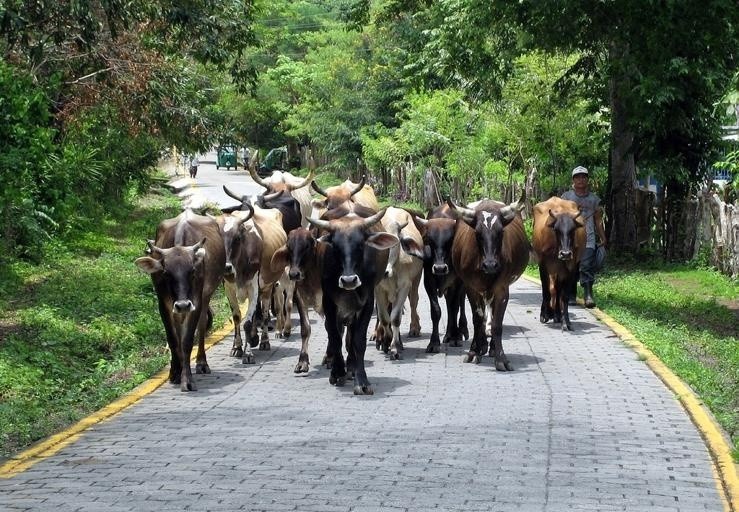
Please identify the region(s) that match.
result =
[216,144,237,170]
[258,147,289,178]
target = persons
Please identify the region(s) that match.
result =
[558,164,608,310]
[192,156,200,175]
[237,146,252,170]
[187,155,197,179]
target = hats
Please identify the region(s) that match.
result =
[572,165,589,181]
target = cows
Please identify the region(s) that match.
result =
[200,150,532,394]
[532,196,589,332]
[134,208,226,392]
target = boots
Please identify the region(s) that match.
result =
[584,281,596,308]
[568,282,577,306]
